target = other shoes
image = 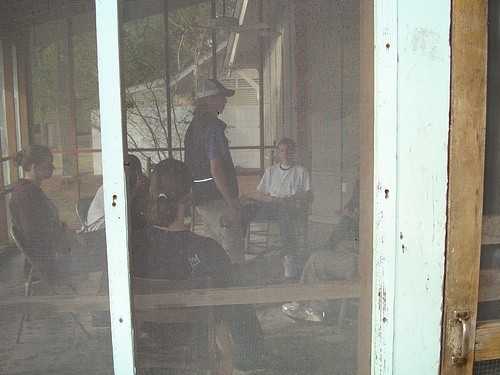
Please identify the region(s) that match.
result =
[283,255,296,277]
[281,300,325,323]
[90,311,111,327]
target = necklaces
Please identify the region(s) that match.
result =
[278,162,294,171]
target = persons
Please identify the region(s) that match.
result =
[9,78,264,375]
[239,136,314,280]
[279,168,360,326]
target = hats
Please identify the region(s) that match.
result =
[192,79,235,100]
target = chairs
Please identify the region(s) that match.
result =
[10,223,95,344]
[75,197,93,225]
[132,278,216,375]
[245,215,307,255]
